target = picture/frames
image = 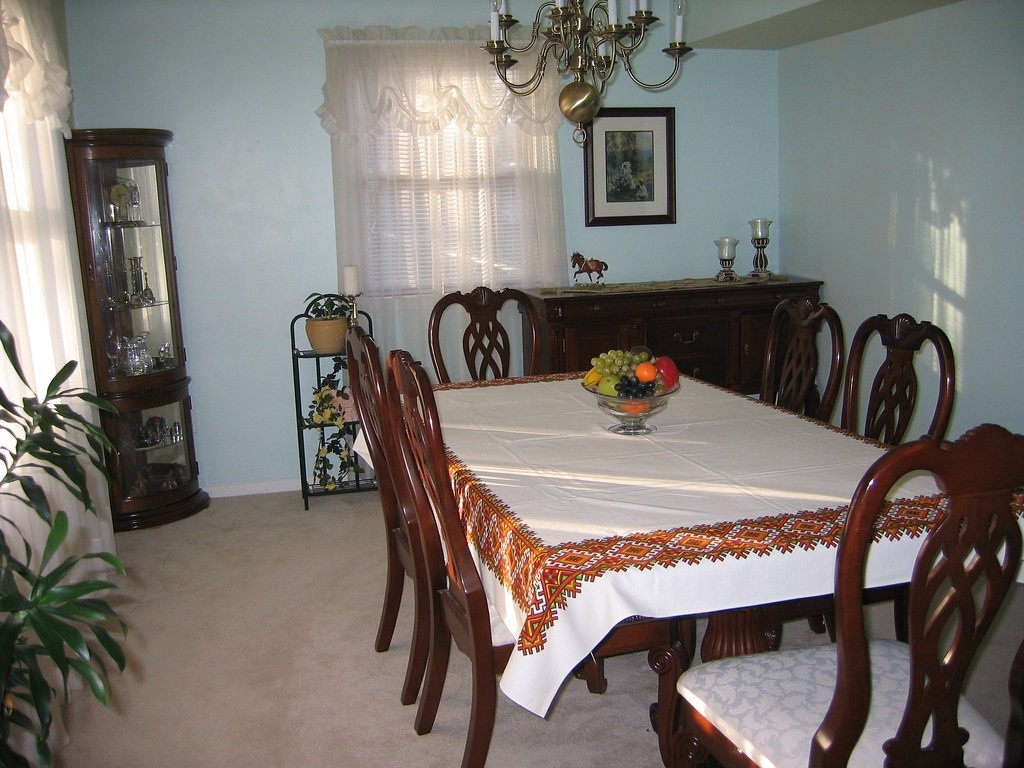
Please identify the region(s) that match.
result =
[584,106,676,228]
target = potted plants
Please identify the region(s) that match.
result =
[303,292,349,353]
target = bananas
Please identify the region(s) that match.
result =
[583,366,602,387]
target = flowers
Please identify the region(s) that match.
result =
[308,356,365,497]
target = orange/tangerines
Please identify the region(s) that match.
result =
[635,362,657,382]
[620,400,651,412]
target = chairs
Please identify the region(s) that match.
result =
[759,296,846,425]
[675,425,1024,768]
[808,314,956,646]
[343,325,585,706]
[427,287,542,384]
[383,349,689,768]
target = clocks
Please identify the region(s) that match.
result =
[102,177,147,227]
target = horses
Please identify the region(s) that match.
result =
[570,251,608,285]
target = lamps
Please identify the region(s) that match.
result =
[479,0,693,143]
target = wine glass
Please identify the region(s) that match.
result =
[104,331,154,376]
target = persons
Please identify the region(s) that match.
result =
[636,179,648,199]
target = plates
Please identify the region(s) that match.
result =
[134,440,163,452]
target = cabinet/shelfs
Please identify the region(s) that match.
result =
[290,294,378,510]
[516,275,825,416]
[61,129,211,532]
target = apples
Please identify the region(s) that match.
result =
[597,374,621,396]
[653,356,679,390]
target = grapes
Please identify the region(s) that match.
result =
[614,375,656,400]
[590,349,650,379]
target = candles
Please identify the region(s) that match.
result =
[747,218,773,238]
[713,236,741,260]
[341,264,359,297]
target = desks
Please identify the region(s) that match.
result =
[351,372,1024,768]
